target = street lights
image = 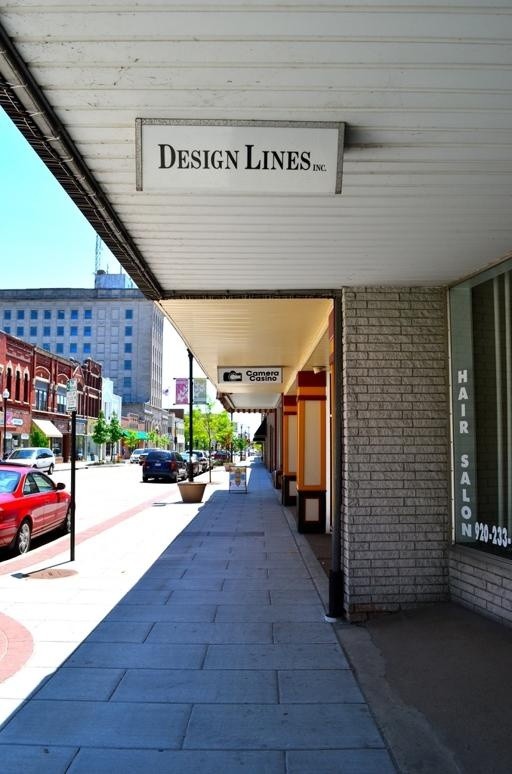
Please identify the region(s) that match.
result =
[1,388,10,457]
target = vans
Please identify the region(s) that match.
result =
[6,447,55,475]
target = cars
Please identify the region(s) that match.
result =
[0,464,72,555]
[130,448,231,483]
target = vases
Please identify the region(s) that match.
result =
[178,483,207,502]
